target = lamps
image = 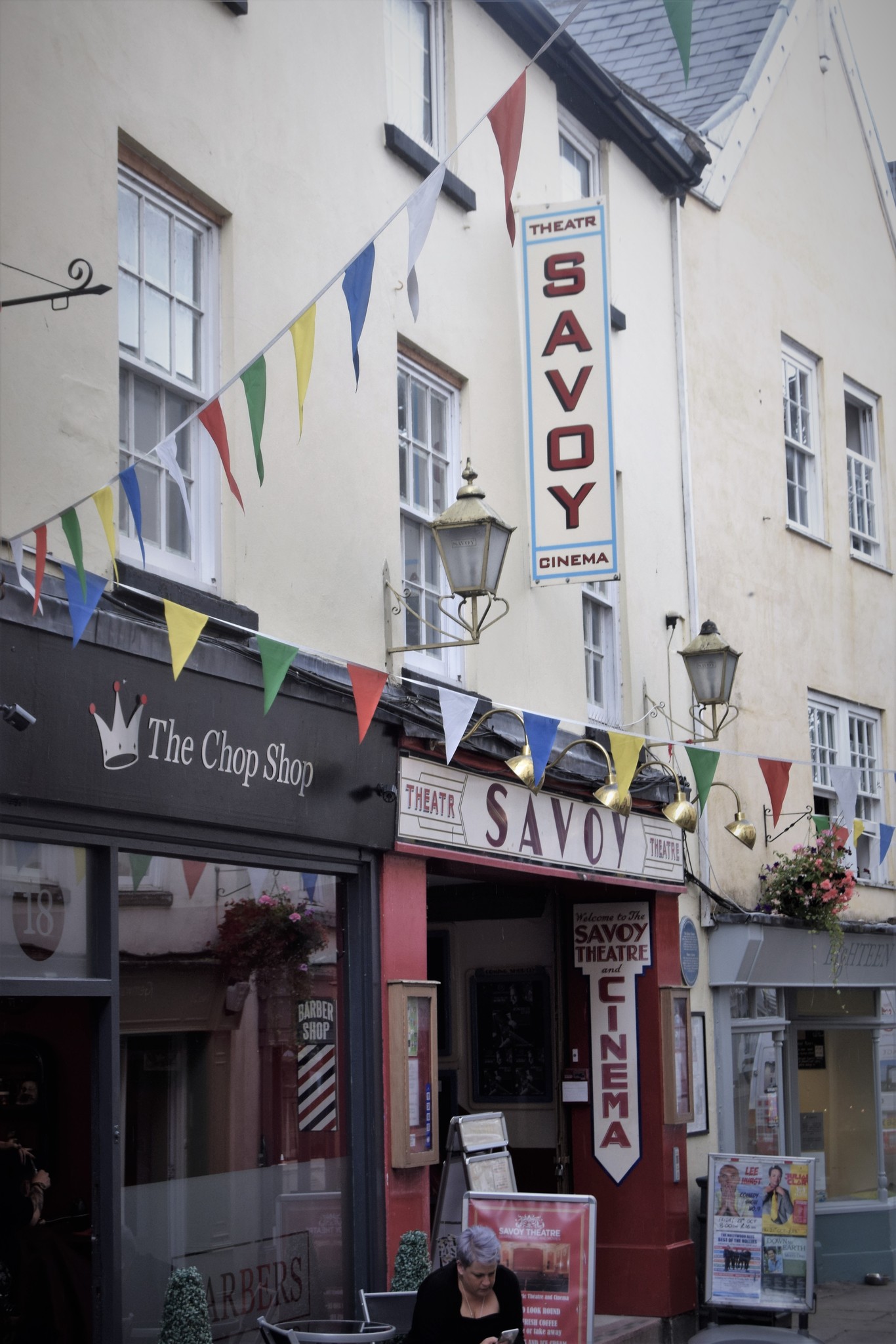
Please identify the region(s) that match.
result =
[382,457,519,684]
[545,738,632,817]
[633,761,697,834]
[643,618,744,768]
[689,782,756,849]
[426,708,547,797]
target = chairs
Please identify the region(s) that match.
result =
[257,1289,417,1344]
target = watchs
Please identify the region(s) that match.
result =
[30,1182,47,1191]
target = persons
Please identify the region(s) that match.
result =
[723,1245,751,1271]
[0,1080,53,1322]
[713,1164,754,1218]
[759,1165,794,1225]
[767,1249,783,1274]
[402,1225,525,1344]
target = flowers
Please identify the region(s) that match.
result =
[758,810,859,1018]
[205,883,331,1048]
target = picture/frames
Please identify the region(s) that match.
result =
[686,1011,710,1137]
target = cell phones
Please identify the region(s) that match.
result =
[498,1328,520,1344]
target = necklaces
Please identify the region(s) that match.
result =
[460,1279,486,1319]
